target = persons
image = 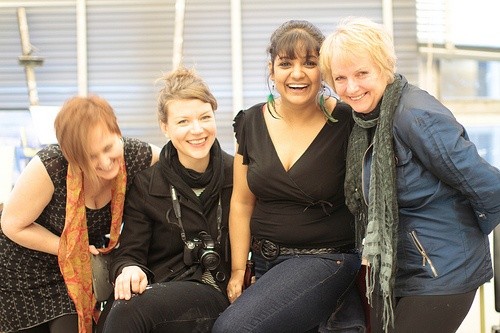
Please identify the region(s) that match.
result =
[318,16,500,333]
[0,95,162,333]
[96,67,234,333]
[211,20,363,333]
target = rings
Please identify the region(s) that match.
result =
[226,292,233,299]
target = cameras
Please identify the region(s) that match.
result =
[184,231,223,270]
